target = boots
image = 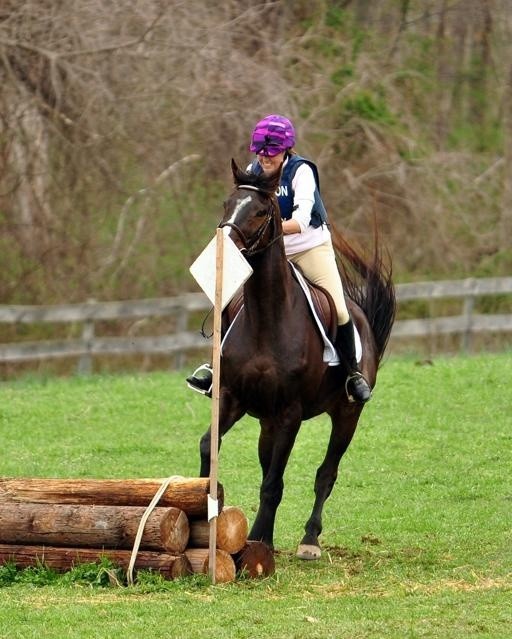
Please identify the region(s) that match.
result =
[335,311,372,403]
[185,365,212,398]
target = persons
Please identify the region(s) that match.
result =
[184,114,373,408]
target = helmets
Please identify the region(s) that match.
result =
[248,113,296,157]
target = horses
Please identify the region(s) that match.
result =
[199,156,397,559]
